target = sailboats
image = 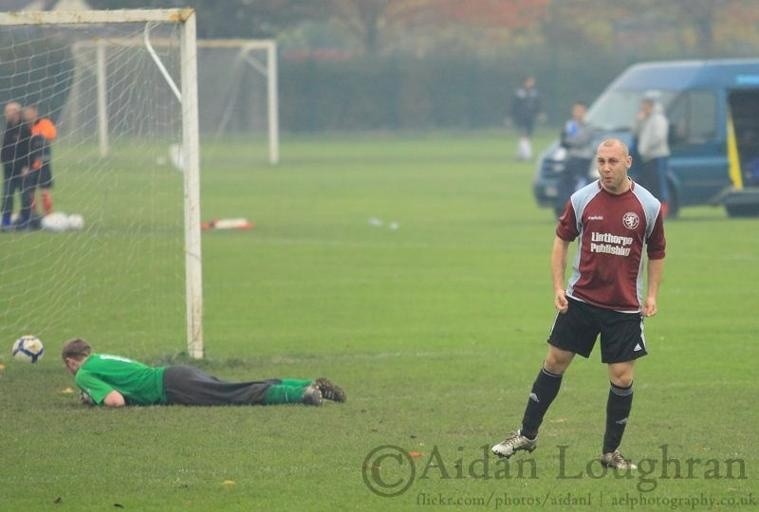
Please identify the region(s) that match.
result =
[40,213,66,233]
[69,212,86,232]
[11,334,42,363]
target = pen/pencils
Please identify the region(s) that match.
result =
[534,58,758,221]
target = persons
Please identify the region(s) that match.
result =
[633,99,670,219]
[62,336,346,409]
[510,75,545,161]
[491,137,666,470]
[556,102,595,220]
[10,106,57,230]
[1,101,41,230]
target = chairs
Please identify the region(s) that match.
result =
[302,384,324,406]
[491,429,540,459]
[601,451,637,471]
[318,377,348,404]
